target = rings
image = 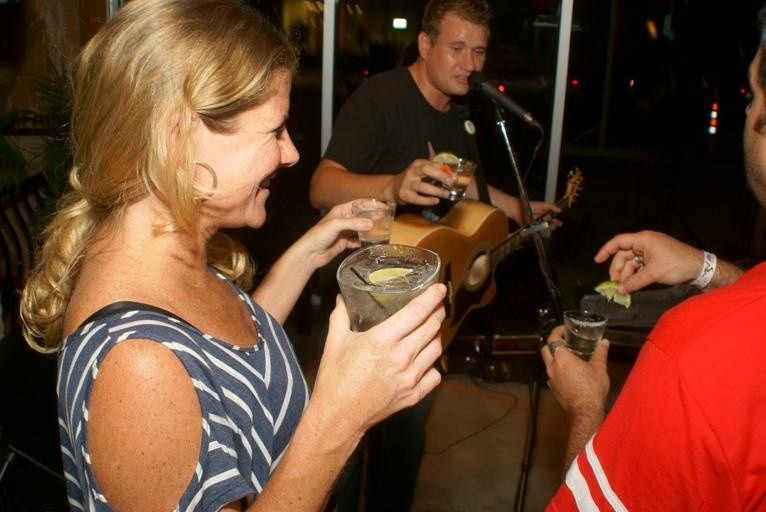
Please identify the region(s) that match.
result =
[633,254,641,263]
[549,339,569,354]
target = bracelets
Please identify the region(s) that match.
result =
[690,248,718,290]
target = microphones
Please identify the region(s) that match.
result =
[468,71,539,130]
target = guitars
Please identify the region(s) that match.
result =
[388,168,582,354]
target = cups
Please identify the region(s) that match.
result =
[563,310,608,360]
[442,158,477,201]
[336,199,441,331]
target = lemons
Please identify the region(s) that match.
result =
[368,268,413,284]
[433,153,461,165]
[593,280,631,310]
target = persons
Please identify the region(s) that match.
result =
[19,0,450,512]
[307,0,563,232]
[539,4,766,512]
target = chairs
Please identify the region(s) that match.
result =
[0,172,65,486]
